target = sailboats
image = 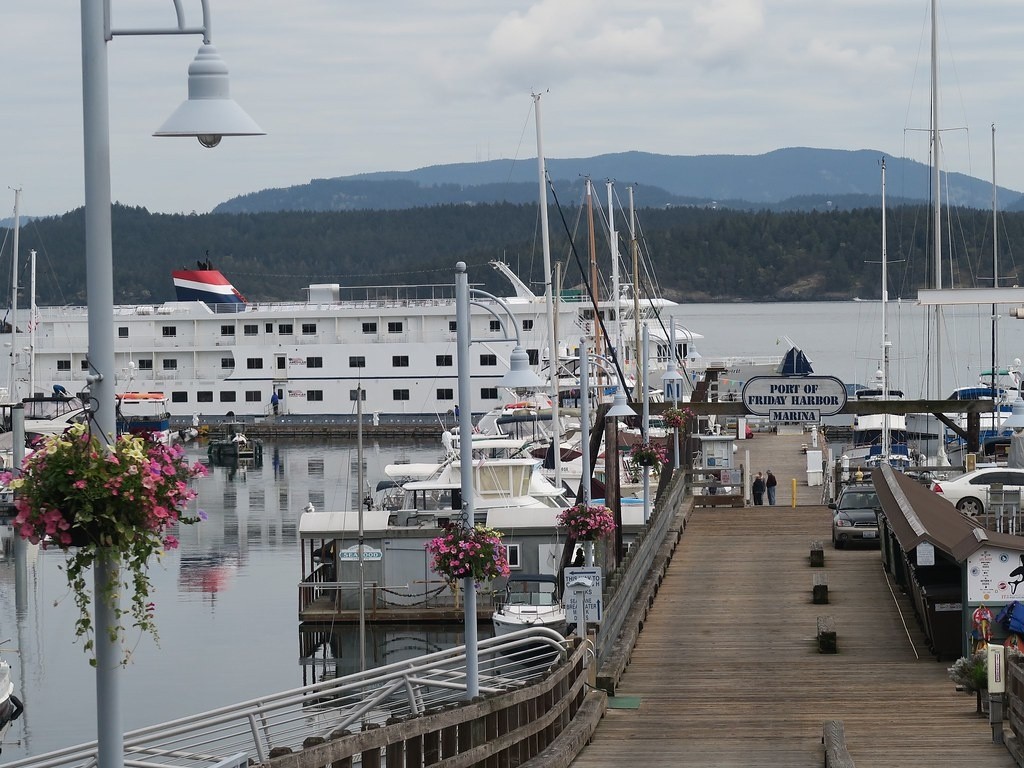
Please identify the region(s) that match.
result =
[0,1,1024,623]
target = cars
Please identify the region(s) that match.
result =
[927,467,1024,517]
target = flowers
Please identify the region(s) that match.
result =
[423,522,511,624]
[662,407,695,446]
[630,438,670,475]
[2,421,209,670]
[555,504,619,562]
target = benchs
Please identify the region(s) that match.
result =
[812,572,829,604]
[821,720,856,768]
[809,539,824,567]
[802,446,809,455]
[817,615,837,653]
[802,443,807,447]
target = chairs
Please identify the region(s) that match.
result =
[868,495,879,506]
[846,494,857,506]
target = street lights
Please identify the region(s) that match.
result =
[669,313,704,469]
[75,0,267,768]
[640,320,684,529]
[450,259,552,698]
[576,335,639,568]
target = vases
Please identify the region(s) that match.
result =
[578,527,596,542]
[455,562,473,580]
[639,458,653,466]
[55,509,112,548]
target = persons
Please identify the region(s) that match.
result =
[913,451,927,467]
[271,392,279,415]
[455,405,460,422]
[752,470,777,505]
[573,548,585,567]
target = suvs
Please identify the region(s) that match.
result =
[827,480,885,549]
